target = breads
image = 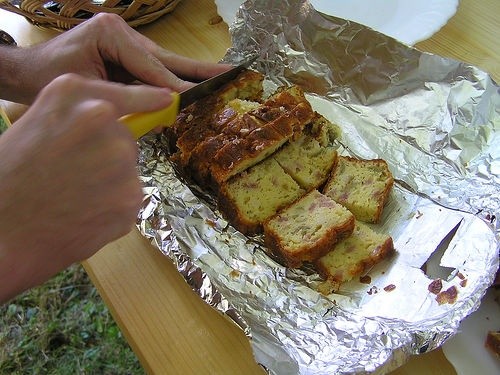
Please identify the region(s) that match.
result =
[219,157,303,234]
[263,190,354,266]
[162,66,315,185]
[274,133,339,190]
[318,220,392,283]
[325,153,392,221]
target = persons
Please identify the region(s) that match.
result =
[0,12,236,307]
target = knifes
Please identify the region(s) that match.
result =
[115,54,258,139]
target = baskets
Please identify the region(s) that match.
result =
[1,1,179,34]
